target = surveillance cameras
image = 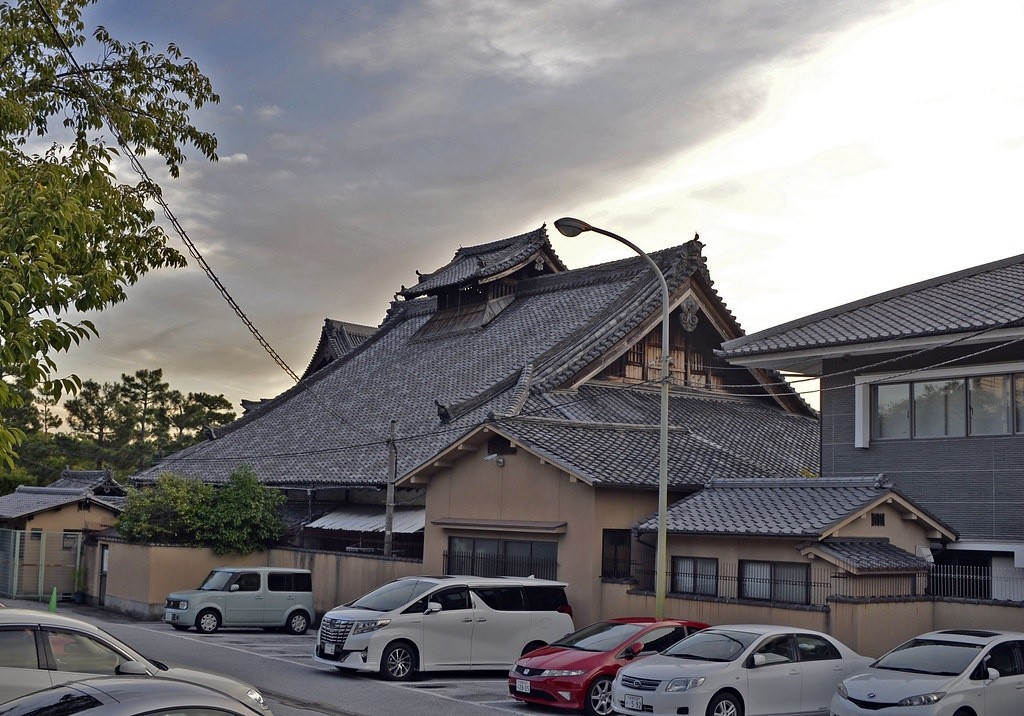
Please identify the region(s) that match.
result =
[484,454,498,461]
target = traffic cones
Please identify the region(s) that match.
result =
[47,586,59,613]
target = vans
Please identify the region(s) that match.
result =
[313,575,577,682]
[161,565,315,636]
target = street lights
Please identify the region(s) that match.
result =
[554,215,670,618]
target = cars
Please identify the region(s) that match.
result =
[506,616,716,716]
[0,676,265,716]
[829,627,1023,716]
[609,623,877,716]
[0,601,275,715]
